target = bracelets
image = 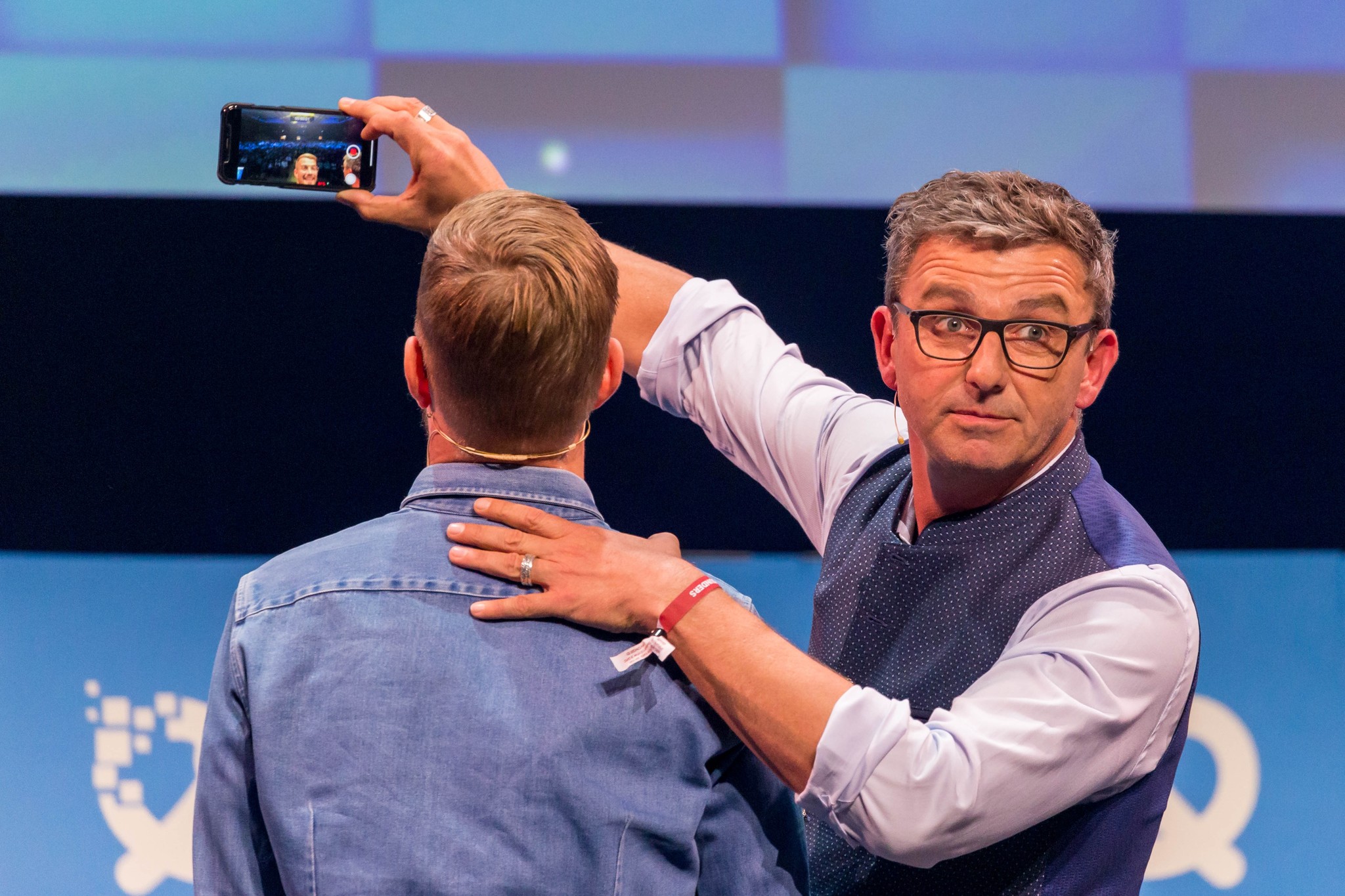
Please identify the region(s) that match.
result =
[609,575,722,672]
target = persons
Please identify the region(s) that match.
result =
[192,188,809,896]
[336,152,362,188]
[293,153,319,185]
[334,94,1201,896]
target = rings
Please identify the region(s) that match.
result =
[520,553,536,586]
[414,105,437,123]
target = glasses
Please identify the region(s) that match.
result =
[341,165,347,170]
[890,302,1098,369]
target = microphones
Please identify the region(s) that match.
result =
[894,392,904,444]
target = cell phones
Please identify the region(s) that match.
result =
[216,103,380,192]
